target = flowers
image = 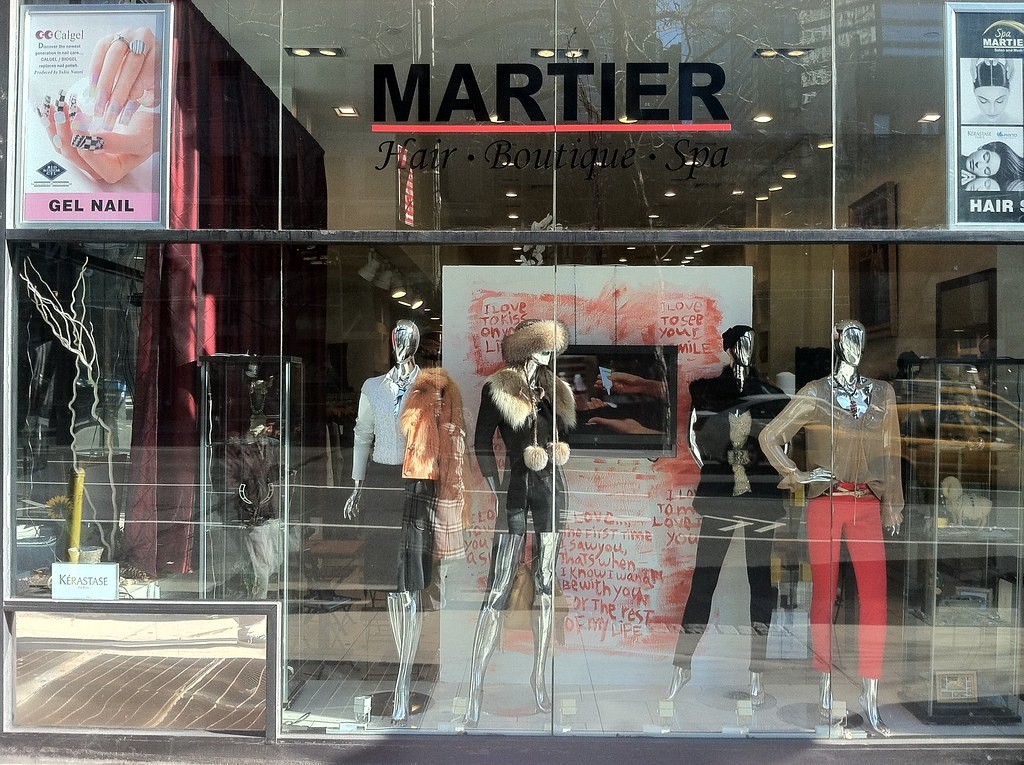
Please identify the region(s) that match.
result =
[46,496,83,551]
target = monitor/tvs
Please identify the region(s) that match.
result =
[556,345,678,458]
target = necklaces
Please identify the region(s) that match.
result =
[833,375,861,419]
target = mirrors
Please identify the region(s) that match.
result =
[935,266,998,466]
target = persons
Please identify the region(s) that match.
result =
[960,142,1024,190]
[209,416,285,649]
[343,320,464,724]
[758,319,904,738]
[587,372,666,434]
[973,57,1013,123]
[660,325,792,709]
[463,319,577,727]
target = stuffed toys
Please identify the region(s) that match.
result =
[942,475,993,532]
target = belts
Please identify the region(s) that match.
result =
[822,484,877,497]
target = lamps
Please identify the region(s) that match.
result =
[358,246,424,309]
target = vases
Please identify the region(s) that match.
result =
[67,546,104,564]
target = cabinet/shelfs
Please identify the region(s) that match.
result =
[895,358,1023,727]
[198,354,305,710]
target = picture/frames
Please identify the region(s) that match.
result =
[847,181,896,340]
[943,1,1024,232]
[15,2,174,231]
